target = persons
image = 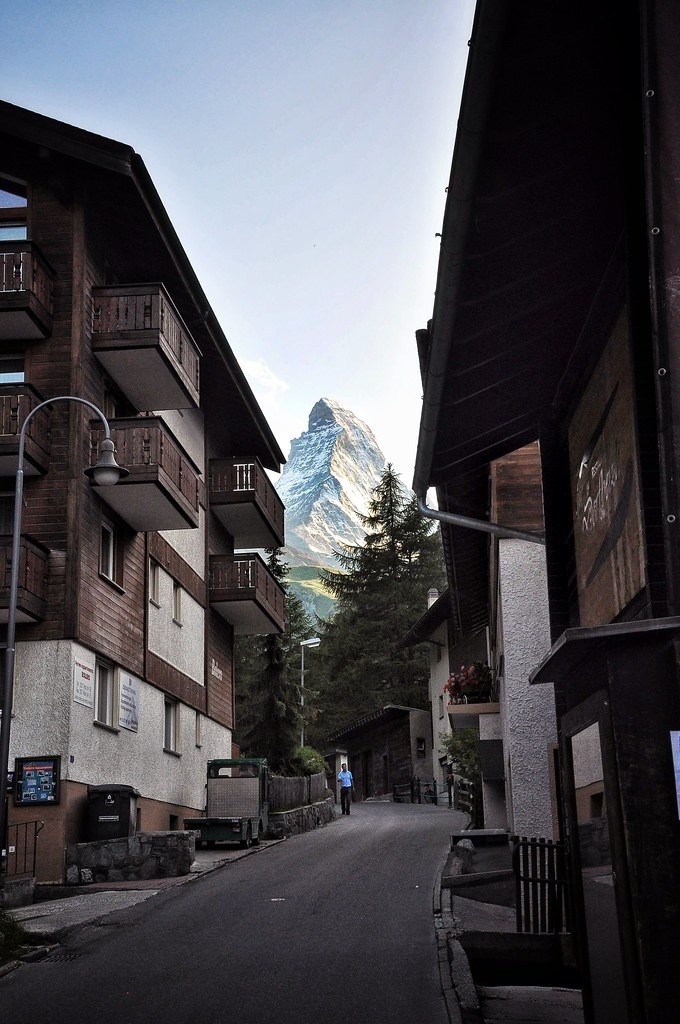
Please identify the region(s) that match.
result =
[337,763,356,815]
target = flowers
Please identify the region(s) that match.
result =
[443,665,489,705]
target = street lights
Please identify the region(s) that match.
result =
[0,395,132,912]
[299,638,322,748]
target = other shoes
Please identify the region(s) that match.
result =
[342,811,345,815]
[346,812,350,815]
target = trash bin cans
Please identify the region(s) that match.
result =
[86,784,140,838]
